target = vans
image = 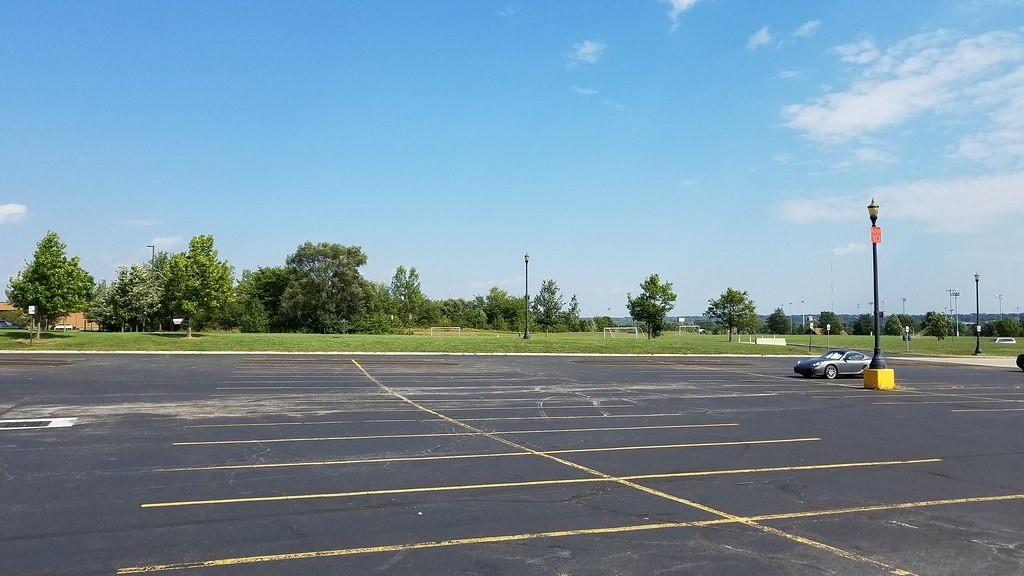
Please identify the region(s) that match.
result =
[988,337,1017,344]
[51,323,73,330]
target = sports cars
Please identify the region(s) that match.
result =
[793,349,873,379]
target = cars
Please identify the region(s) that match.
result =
[0,319,23,329]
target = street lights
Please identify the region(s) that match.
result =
[868,301,874,315]
[1016,307,1022,325]
[945,288,956,321]
[789,303,794,335]
[994,294,1004,323]
[974,273,983,355]
[879,300,888,315]
[951,292,962,337]
[902,297,909,316]
[866,197,895,390]
[146,245,154,276]
[801,300,805,331]
[524,252,532,339]
